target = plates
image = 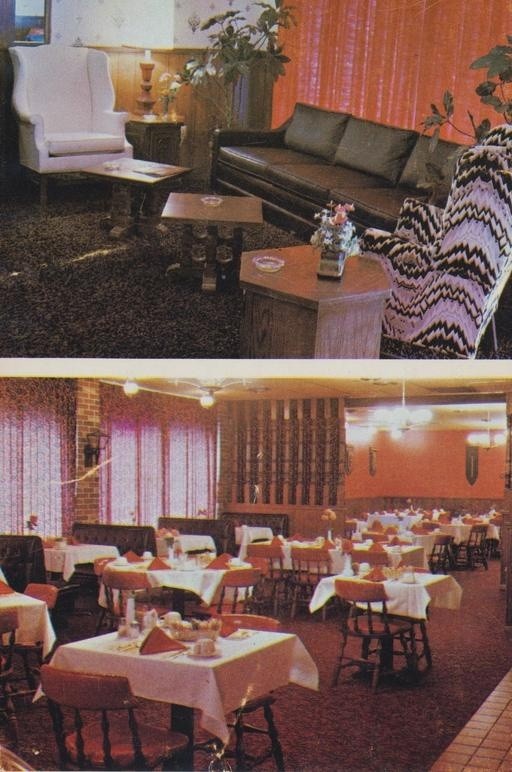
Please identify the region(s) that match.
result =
[112,563,130,566]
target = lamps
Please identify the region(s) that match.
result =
[83,425,113,471]
[198,386,221,412]
[123,378,140,402]
[368,380,437,441]
[465,403,507,457]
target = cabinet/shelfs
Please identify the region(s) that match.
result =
[122,111,189,207]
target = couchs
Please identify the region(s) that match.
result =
[203,94,471,269]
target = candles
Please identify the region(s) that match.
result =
[142,47,154,64]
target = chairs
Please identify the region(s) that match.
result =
[2,491,511,771]
[5,39,138,212]
[355,118,511,358]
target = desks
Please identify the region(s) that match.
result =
[232,243,397,360]
[78,155,194,246]
[159,191,264,296]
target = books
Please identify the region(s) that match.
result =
[133,166,178,176]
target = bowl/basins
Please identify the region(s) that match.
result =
[253,257,284,274]
[143,115,157,121]
[201,197,224,208]
[104,161,121,170]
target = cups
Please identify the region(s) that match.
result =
[229,558,240,565]
[195,554,205,566]
[119,610,222,660]
[118,557,128,563]
[358,563,371,574]
[55,541,65,549]
[144,552,152,559]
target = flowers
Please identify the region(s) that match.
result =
[158,2,305,132]
[309,198,365,262]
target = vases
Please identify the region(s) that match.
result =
[316,250,346,281]
